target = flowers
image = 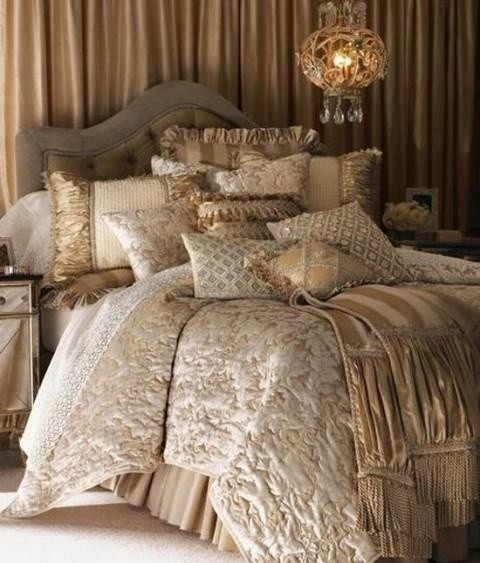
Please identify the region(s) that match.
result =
[382,199,430,232]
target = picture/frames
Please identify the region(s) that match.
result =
[406,185,439,217]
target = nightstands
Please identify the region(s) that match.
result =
[0,276,44,464]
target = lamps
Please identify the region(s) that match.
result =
[294,0,390,124]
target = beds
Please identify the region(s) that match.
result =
[12,73,480,563]
